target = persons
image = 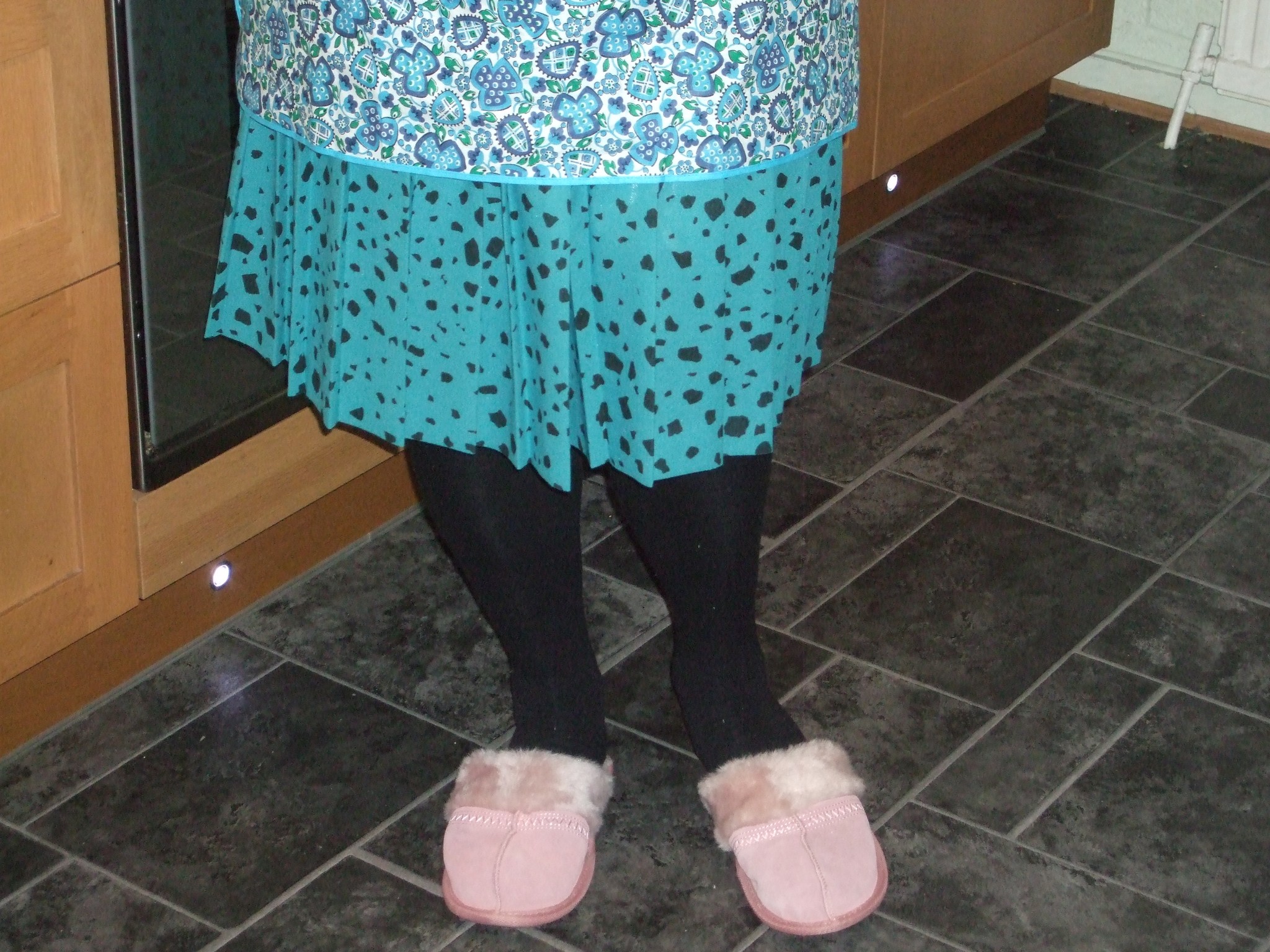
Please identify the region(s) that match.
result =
[202,0,889,937]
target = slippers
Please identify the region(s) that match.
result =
[700,737,889,937]
[443,748,615,927]
